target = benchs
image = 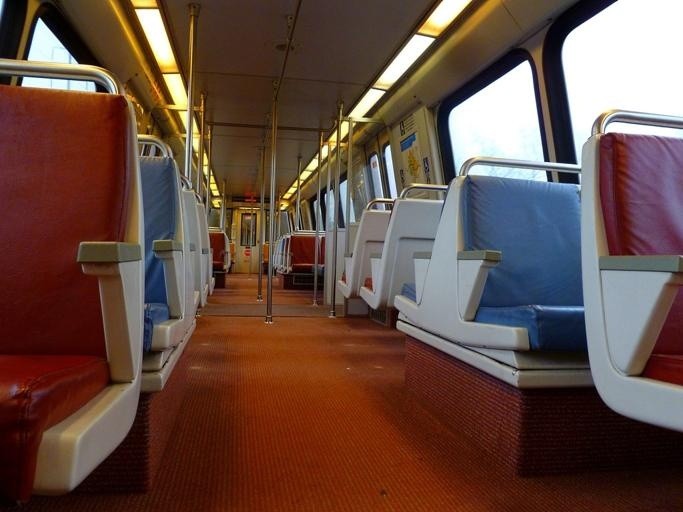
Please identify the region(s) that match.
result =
[393,156,594,387]
[581,108,682,432]
[264,156,448,326]
[1,58,146,494]
[136,133,233,392]
